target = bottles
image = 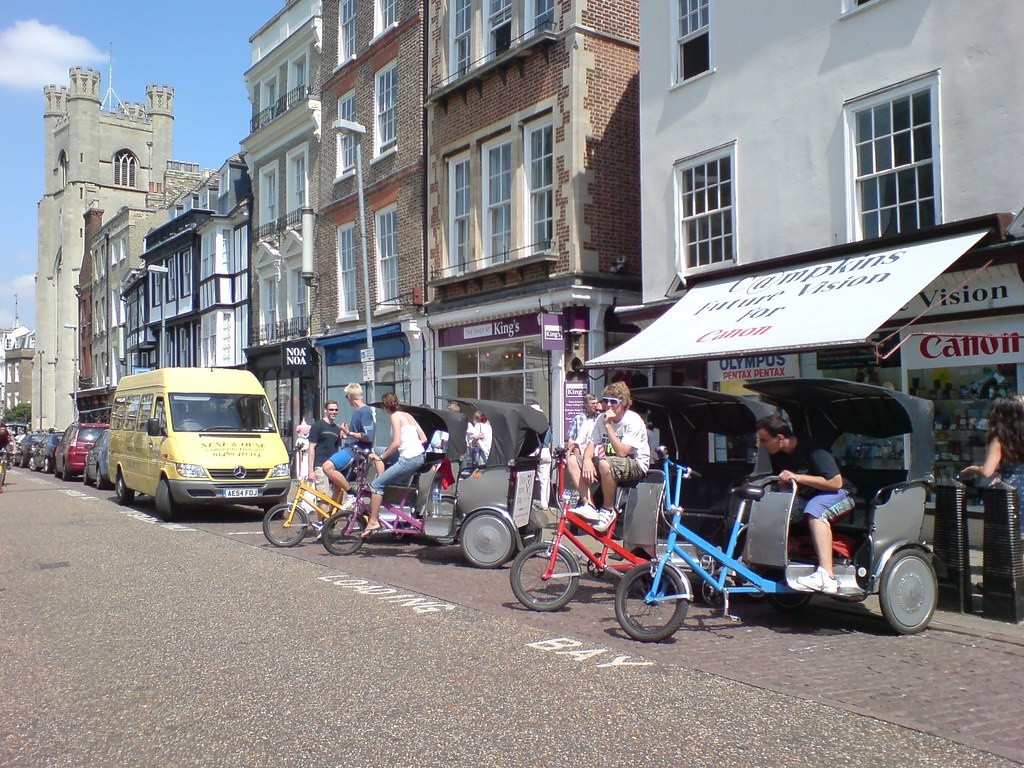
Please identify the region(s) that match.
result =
[431,484,441,518]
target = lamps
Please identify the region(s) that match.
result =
[118,323,126,366]
[301,209,320,295]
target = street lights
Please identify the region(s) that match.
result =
[147,266,169,370]
[332,118,377,421]
[37,351,46,433]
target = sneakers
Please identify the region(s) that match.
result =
[568,504,599,522]
[796,566,837,593]
[593,508,616,531]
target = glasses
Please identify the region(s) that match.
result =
[329,409,338,411]
[603,397,622,405]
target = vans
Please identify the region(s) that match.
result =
[106,366,293,518]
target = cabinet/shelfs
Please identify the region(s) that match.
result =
[927,398,993,462]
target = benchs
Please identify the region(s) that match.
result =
[681,461,756,515]
[442,455,541,502]
[392,452,462,490]
[831,469,929,535]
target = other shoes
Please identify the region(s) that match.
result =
[6,464,11,470]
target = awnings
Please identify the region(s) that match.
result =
[575,227,996,370]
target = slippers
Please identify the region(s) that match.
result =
[360,526,381,538]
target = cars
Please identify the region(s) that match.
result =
[83,430,115,489]
[28,434,66,472]
[12,432,48,467]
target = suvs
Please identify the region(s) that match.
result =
[53,422,111,478]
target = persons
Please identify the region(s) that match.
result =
[960,396,1024,590]
[361,392,428,539]
[757,416,857,593]
[307,400,342,537]
[310,383,375,531]
[425,426,449,502]
[284,417,317,522]
[568,380,650,532]
[448,402,492,470]
[529,404,554,510]
[0,423,16,470]
[568,393,660,471]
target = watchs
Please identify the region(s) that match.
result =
[379,455,383,460]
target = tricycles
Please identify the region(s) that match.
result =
[614,374,947,639]
[508,385,787,612]
[259,396,550,569]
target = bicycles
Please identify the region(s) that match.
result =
[0,450,11,487]
